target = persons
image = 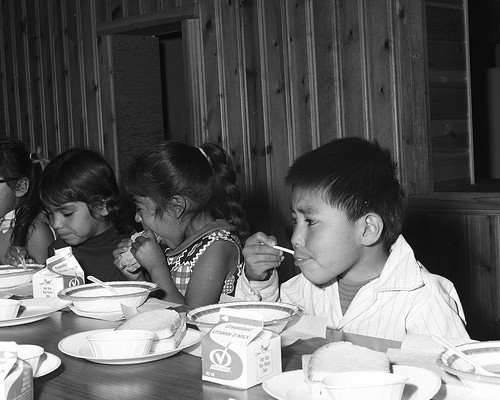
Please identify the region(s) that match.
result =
[38,148,136,282]
[0,136,59,269]
[119,140,249,308]
[236,137,470,345]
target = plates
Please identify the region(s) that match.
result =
[58,328,205,365]
[262,364,442,400]
[33,352,61,380]
[0,298,72,327]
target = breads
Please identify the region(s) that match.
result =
[300,340,390,384]
[114,308,186,351]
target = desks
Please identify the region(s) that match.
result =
[1,306,402,400]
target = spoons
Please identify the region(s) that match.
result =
[432,334,499,376]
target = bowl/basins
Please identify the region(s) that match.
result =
[320,370,410,400]
[182,300,306,334]
[436,339,500,399]
[84,330,157,358]
[57,281,161,313]
[16,344,44,376]
[0,263,45,289]
[0,298,23,320]
